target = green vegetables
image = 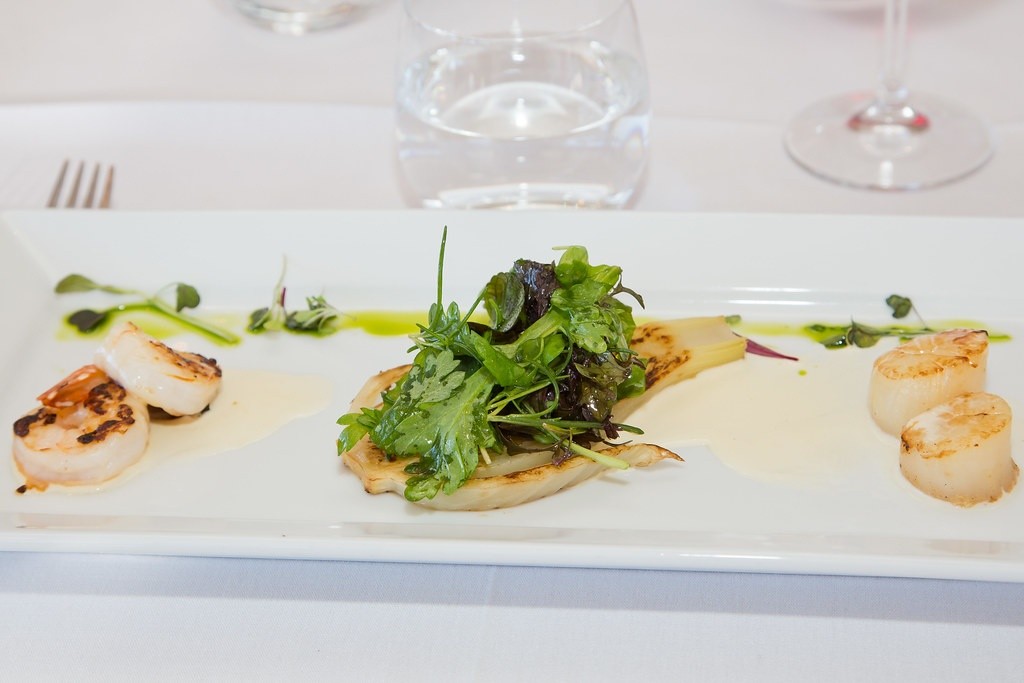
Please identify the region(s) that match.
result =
[52,226,938,505]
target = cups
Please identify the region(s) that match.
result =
[394,1,652,209]
[237,1,386,32]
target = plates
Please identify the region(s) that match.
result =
[3,211,1023,583]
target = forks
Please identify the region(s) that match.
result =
[44,160,116,207]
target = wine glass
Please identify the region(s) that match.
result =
[784,1,998,191]
